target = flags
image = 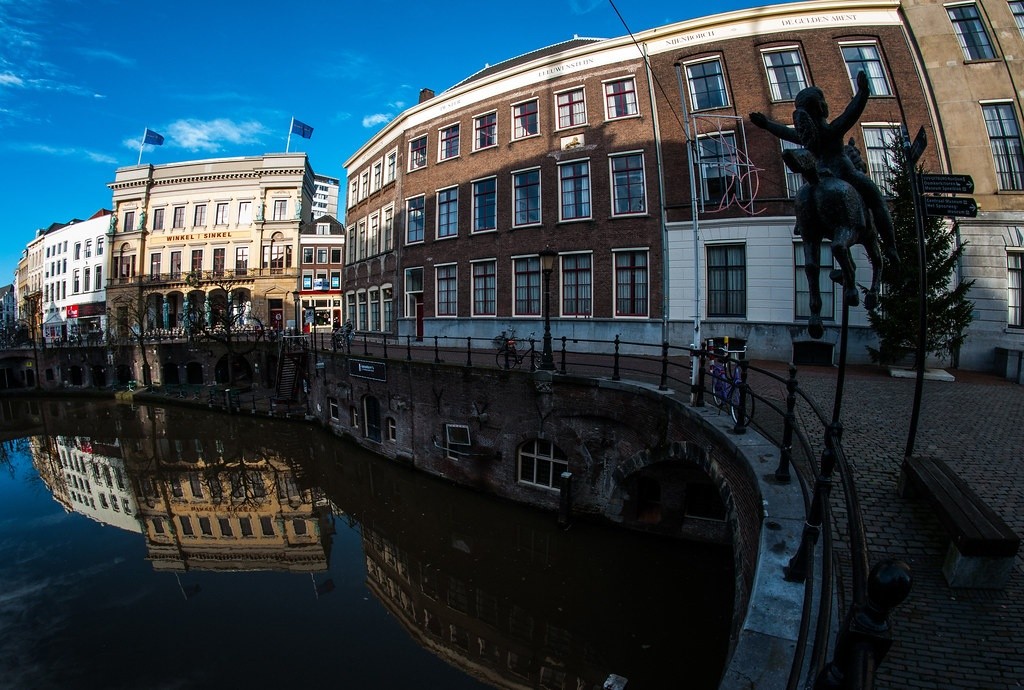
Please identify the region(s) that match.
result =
[291,119,314,139]
[144,129,164,146]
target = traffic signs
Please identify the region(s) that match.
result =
[920,196,980,218]
[907,124,930,165]
[918,172,976,193]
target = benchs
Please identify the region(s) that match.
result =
[902,455,1020,588]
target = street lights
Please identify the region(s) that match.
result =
[537,244,558,370]
[291,288,300,338]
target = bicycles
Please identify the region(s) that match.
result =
[711,343,757,427]
[492,327,525,352]
[496,332,544,371]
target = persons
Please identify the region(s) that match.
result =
[77,334,83,346]
[341,319,352,338]
[99,328,104,344]
[10,333,17,347]
[749,72,901,340]
